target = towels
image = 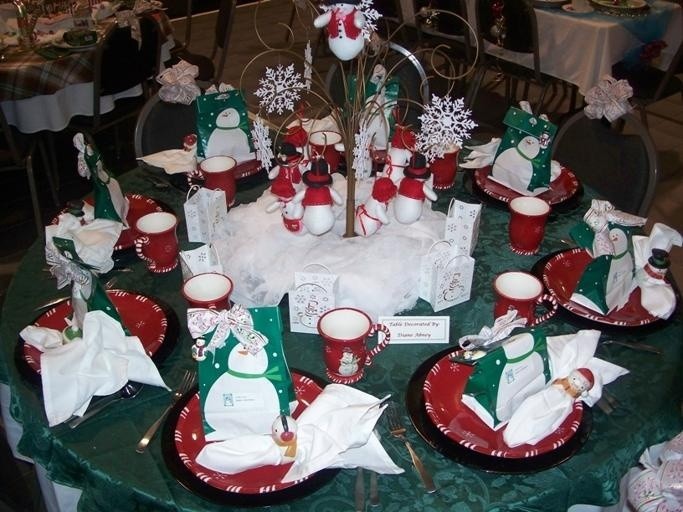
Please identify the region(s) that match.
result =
[192,369,405,491]
[609,221,682,322]
[499,325,632,453]
[15,305,172,431]
[454,133,563,183]
[41,208,123,276]
[133,149,203,176]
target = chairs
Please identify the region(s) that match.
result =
[320,34,433,130]
[0,98,59,274]
[548,92,661,220]
[284,0,579,125]
[75,0,237,174]
[606,31,682,134]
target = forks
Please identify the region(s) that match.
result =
[133,370,198,454]
[32,276,118,312]
[381,400,436,493]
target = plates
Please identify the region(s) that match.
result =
[47,192,176,271]
[180,157,264,191]
[472,158,584,214]
[531,249,681,335]
[561,4,594,15]
[406,342,593,474]
[159,368,343,507]
[50,37,100,50]
[335,147,386,173]
[13,289,180,388]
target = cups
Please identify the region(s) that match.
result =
[181,272,235,312]
[136,212,179,274]
[507,196,551,256]
[495,272,557,330]
[309,130,343,174]
[571,0,589,11]
[425,141,460,190]
[200,156,237,207]
[315,307,390,384]
[114,11,133,28]
[0,45,8,63]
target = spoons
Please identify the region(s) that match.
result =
[137,173,169,190]
[67,380,144,430]
